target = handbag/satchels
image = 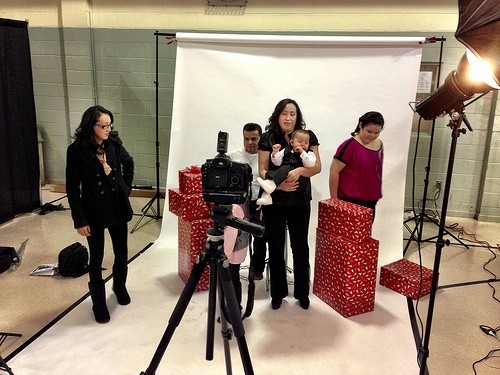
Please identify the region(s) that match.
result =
[58,242,88,277]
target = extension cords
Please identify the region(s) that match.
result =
[411,216,439,222]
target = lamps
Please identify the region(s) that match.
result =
[409,49,500,120]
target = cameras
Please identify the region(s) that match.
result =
[201,131,250,205]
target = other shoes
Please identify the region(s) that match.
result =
[298,295,309,310]
[271,297,282,309]
[254,270,263,280]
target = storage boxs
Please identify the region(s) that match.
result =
[313,227,379,318]
[319,198,373,243]
[179,167,203,194]
[379,259,439,300]
[178,216,214,292]
[169,188,214,221]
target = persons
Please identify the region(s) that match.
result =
[257,99,321,310]
[256,130,317,206]
[227,123,268,280]
[218,204,249,324]
[65,105,134,323]
[329,111,384,223]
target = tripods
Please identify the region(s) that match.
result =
[144,202,269,375]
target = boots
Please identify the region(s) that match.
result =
[113,265,130,305]
[88,279,110,323]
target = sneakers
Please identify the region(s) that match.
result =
[257,176,276,194]
[256,192,273,205]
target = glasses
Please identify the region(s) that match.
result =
[95,123,114,130]
